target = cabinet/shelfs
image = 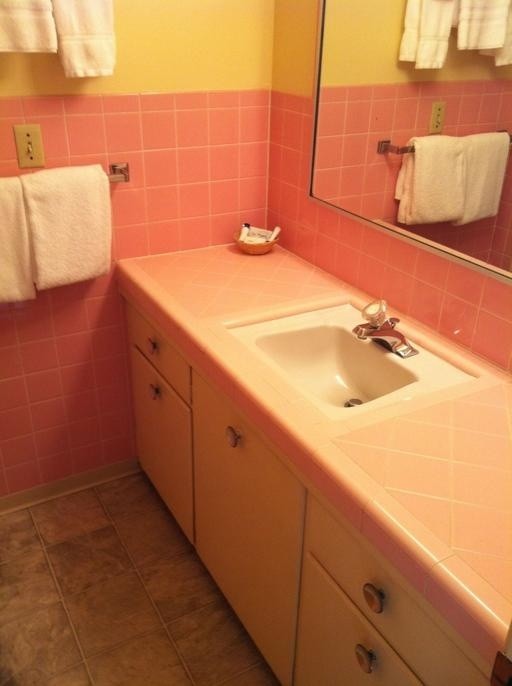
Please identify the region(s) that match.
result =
[124,290,503,686]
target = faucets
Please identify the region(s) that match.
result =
[351,300,419,361]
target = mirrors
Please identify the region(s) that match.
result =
[309,0,511,285]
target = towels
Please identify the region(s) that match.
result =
[1,164,114,302]
[395,133,507,227]
[0,0,115,79]
[399,0,512,76]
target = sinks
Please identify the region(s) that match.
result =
[221,297,480,422]
